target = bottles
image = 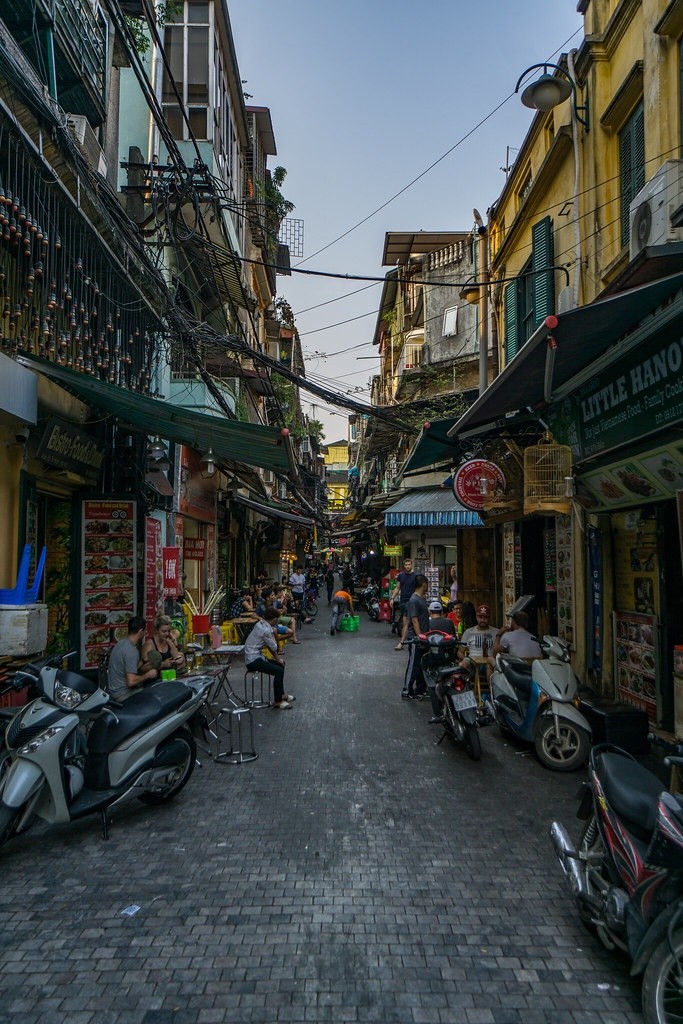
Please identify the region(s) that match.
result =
[483,638,488,657]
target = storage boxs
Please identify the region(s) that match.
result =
[0,603,49,656]
[339,615,360,632]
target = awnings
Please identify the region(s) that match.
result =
[365,269,682,526]
[17,347,313,526]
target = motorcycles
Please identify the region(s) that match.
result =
[400,629,494,761]
[547,731,683,1024]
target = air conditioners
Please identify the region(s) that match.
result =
[626,158,683,263]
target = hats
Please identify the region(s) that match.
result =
[428,602,442,612]
[476,605,491,616]
[506,612,528,625]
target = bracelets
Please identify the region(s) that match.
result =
[496,634,501,637]
[391,598,394,600]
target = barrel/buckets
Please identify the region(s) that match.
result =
[192,614,210,634]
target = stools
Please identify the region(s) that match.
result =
[591,706,650,756]
[242,668,273,708]
[0,544,47,605]
[217,620,291,661]
[582,694,627,724]
[213,706,259,764]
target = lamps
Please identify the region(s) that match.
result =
[227,474,244,498]
[459,274,490,304]
[514,62,591,135]
[200,445,219,473]
[148,433,168,458]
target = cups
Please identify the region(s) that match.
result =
[186,654,194,670]
[195,653,203,666]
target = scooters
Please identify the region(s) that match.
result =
[292,563,380,630]
[0,646,215,848]
[483,635,594,773]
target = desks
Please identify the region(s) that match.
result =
[193,642,245,706]
[183,664,244,747]
[469,654,495,706]
[228,618,258,643]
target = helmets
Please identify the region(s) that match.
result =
[64,765,84,798]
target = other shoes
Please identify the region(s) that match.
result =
[336,629,341,634]
[394,641,404,650]
[293,639,301,644]
[331,628,334,636]
[303,617,316,624]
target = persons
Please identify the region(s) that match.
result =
[390,558,417,649]
[448,600,463,630]
[290,565,306,602]
[245,608,295,709]
[428,602,455,636]
[457,605,499,670]
[229,589,253,618]
[109,616,158,702]
[486,611,541,688]
[330,589,355,636]
[140,615,184,676]
[458,601,477,639]
[308,560,334,605]
[342,567,379,596]
[401,574,429,700]
[255,582,314,644]
[255,571,268,591]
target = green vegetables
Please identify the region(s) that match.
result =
[86,520,133,625]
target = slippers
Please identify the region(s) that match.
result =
[274,700,293,709]
[282,694,296,701]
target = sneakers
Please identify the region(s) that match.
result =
[415,690,430,697]
[402,692,422,700]
